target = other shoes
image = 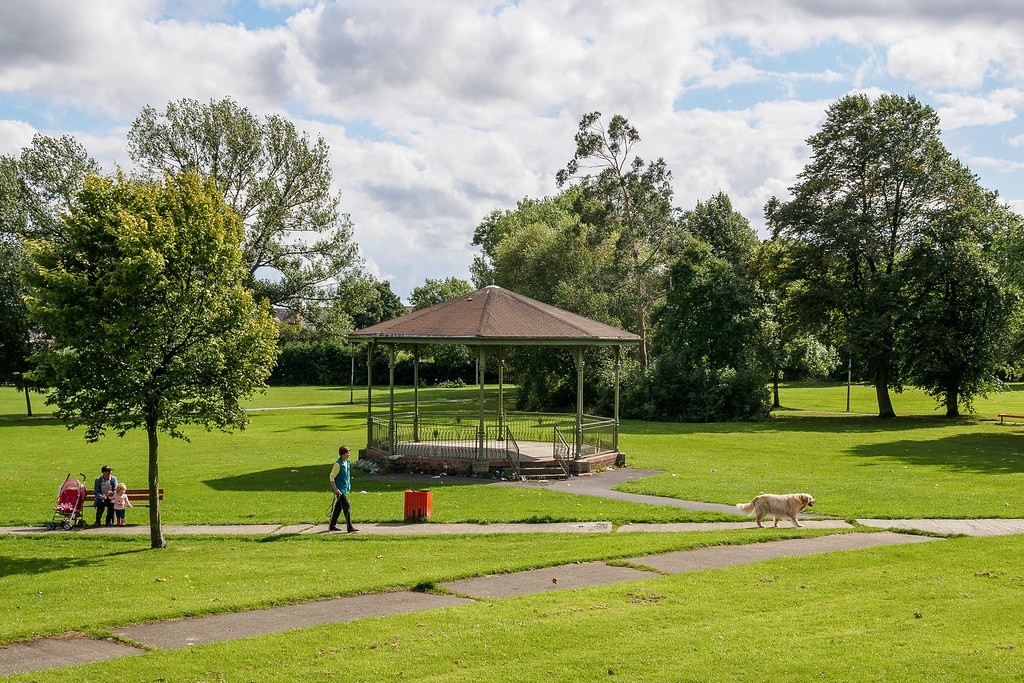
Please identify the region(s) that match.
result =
[121,524,124,527]
[330,526,341,531]
[118,523,120,525]
[94,521,101,527]
[347,527,359,532]
[106,520,110,525]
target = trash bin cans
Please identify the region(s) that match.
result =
[404,489,431,522]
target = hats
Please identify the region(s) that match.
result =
[338,444,350,455]
[102,465,114,472]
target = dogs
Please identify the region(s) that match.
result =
[735,492,817,529]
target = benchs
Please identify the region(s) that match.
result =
[998,413,1024,424]
[75,488,164,525]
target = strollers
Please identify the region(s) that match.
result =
[47,472,87,529]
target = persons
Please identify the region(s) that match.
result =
[329,445,359,531]
[101,482,133,527]
[93,465,118,528]
